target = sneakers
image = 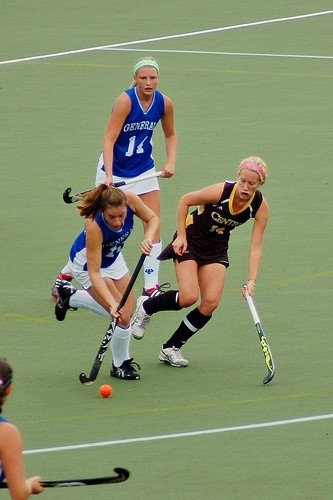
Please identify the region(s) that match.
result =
[142,283,170,298]
[159,344,189,368]
[128,294,152,340]
[50,271,74,302]
[54,280,79,321]
[110,358,140,379]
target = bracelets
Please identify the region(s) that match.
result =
[248,278,256,282]
[246,281,255,286]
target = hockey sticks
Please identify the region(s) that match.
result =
[62,170,167,204]
[0,468,130,489]
[244,284,276,385]
[78,244,152,385]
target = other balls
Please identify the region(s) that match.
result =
[99,384,112,398]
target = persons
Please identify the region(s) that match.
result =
[50,57,177,301]
[131,157,268,367]
[0,357,45,500]
[55,184,160,380]
